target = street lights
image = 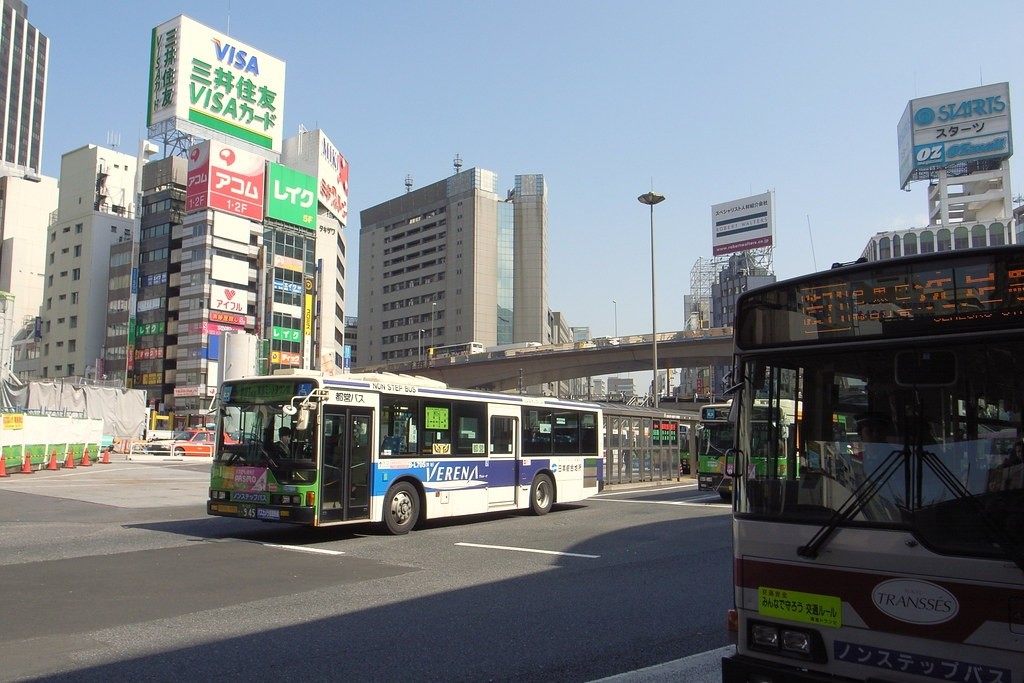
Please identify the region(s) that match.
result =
[431,302,436,347]
[418,329,425,361]
[613,300,617,337]
[638,191,665,409]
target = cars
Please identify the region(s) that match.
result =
[152,429,244,457]
[230,431,260,441]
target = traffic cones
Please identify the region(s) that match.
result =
[45,449,60,471]
[0,453,10,477]
[79,448,94,467]
[98,446,113,464]
[18,451,35,474]
[63,446,76,469]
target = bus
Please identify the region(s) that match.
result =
[719,245,1024,682]
[679,423,699,474]
[430,342,484,367]
[207,368,604,536]
[695,399,846,505]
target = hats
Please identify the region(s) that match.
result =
[279,427,292,436]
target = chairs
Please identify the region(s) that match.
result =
[381,435,405,455]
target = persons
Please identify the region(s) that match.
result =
[852,413,905,479]
[997,441,1024,468]
[275,427,292,458]
